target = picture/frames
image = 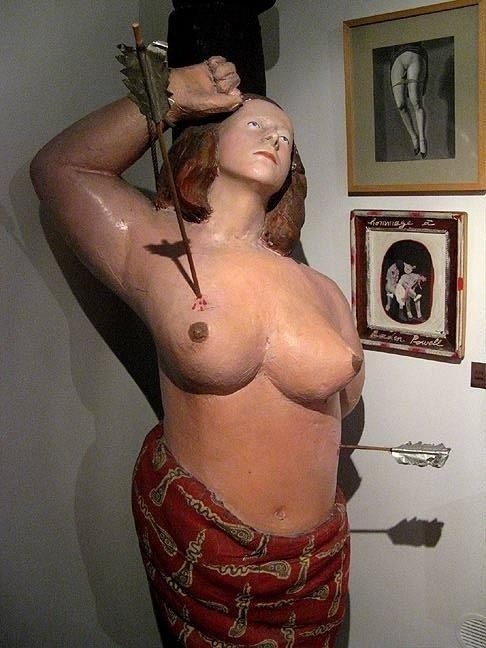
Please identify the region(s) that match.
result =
[336,1,486,367]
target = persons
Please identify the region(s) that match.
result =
[387,43,429,158]
[382,261,429,322]
[24,50,368,647]
